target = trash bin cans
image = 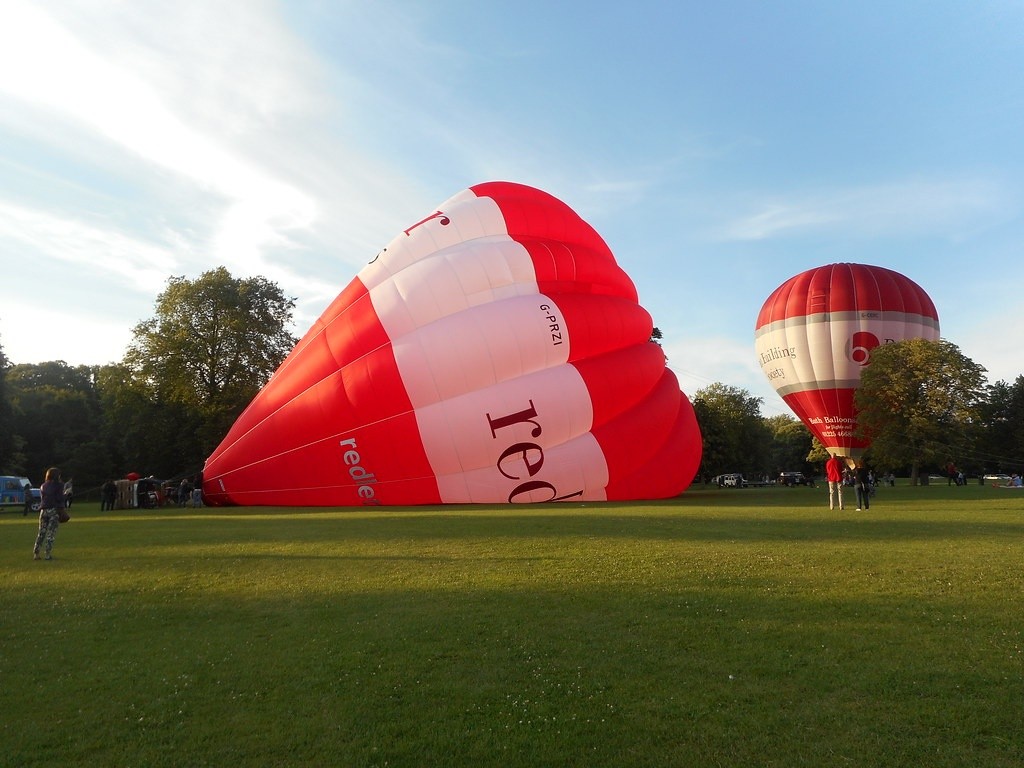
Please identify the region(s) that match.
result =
[920,473,930,486]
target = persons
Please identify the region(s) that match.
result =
[843,474,855,487]
[33,466,65,559]
[791,475,816,488]
[23,484,34,515]
[143,474,175,507]
[100,480,118,511]
[1006,476,1022,486]
[854,456,870,511]
[177,477,191,508]
[867,469,876,496]
[883,473,894,487]
[826,453,844,511]
[760,471,776,487]
[948,461,964,486]
[193,475,202,508]
[736,476,743,488]
[63,476,75,508]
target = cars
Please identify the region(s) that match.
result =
[983,473,1013,480]
[928,473,946,481]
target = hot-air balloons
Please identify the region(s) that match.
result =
[754,262,941,475]
[110,181,705,509]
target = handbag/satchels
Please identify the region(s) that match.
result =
[40,485,70,523]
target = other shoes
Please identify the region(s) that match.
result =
[839,507,844,510]
[34,553,41,560]
[865,509,869,511]
[830,507,834,510]
[45,554,52,560]
[856,509,861,511]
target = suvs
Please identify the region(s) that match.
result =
[711,473,749,488]
[778,471,814,487]
[0,475,43,512]
